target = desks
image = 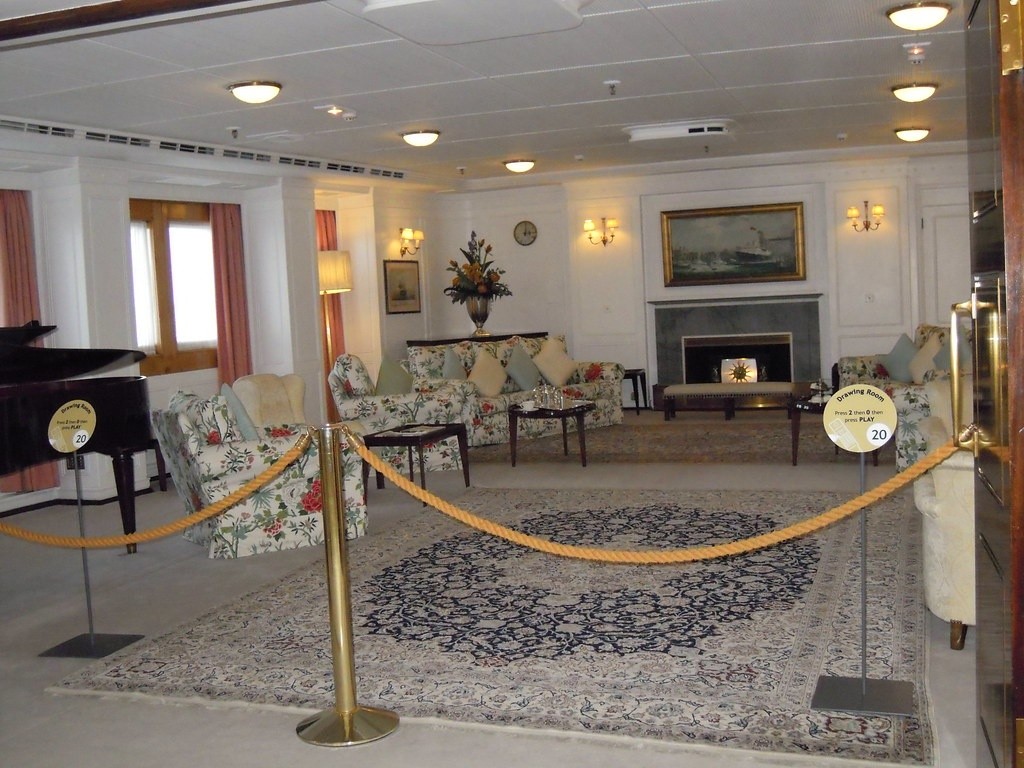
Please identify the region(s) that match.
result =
[361,423,470,508]
[408,332,548,346]
[509,403,596,468]
[623,369,647,417]
[663,382,881,468]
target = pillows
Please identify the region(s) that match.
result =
[932,324,973,371]
[878,333,920,383]
[909,333,943,385]
[445,337,578,399]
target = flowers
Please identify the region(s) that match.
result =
[444,230,514,306]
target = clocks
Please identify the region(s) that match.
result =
[513,221,537,246]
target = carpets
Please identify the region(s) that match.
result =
[466,422,898,466]
[96,488,939,767]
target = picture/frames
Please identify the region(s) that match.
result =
[384,259,422,315]
[660,200,806,288]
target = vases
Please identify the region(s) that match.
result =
[465,295,494,336]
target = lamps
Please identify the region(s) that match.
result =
[582,216,619,248]
[848,0,952,233]
[316,250,352,422]
[400,229,425,257]
[227,81,535,173]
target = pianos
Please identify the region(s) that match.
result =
[0,319,154,554]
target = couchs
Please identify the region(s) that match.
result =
[327,353,463,477]
[152,373,367,559]
[830,324,976,650]
[399,335,627,447]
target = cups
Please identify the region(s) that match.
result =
[809,382,832,403]
[519,401,534,410]
[549,386,575,410]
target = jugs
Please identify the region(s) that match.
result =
[531,379,549,408]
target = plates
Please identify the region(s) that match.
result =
[519,409,539,411]
